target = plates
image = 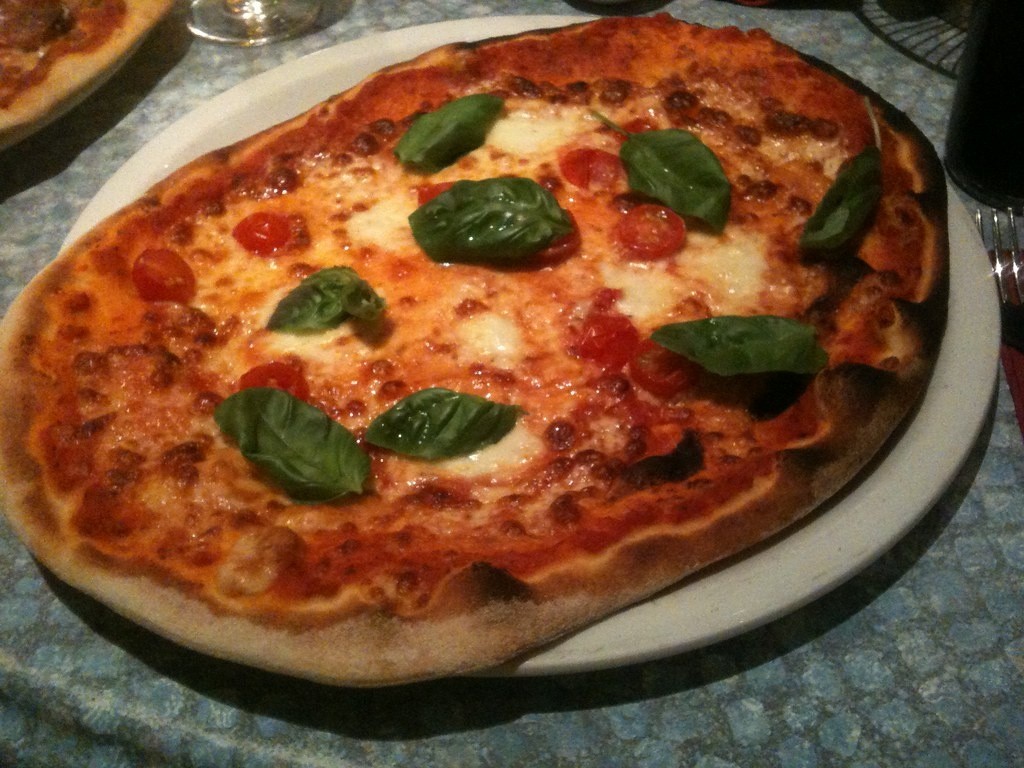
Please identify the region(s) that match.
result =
[58,14,1000,677]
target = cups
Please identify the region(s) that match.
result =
[944,0,1024,212]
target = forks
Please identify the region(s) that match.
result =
[975,207,1024,438]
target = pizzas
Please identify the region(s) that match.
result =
[0,1,173,150]
[3,15,948,685]
